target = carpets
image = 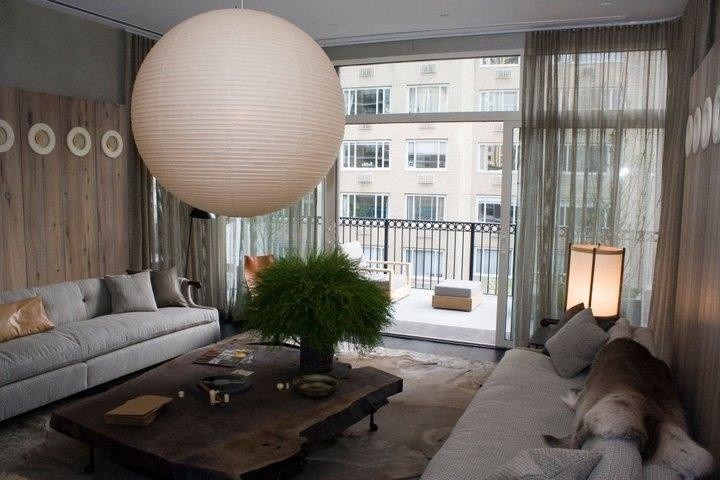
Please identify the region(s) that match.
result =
[0,328,501,480]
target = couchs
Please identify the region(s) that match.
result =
[421,325,720,480]
[1,271,222,421]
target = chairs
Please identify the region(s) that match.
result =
[340,241,412,304]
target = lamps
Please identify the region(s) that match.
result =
[130,0,346,219]
[184,208,216,278]
[563,242,626,323]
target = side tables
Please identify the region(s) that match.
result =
[526,318,556,350]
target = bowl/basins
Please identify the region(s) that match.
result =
[293,374,340,398]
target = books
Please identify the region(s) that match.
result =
[193,346,255,368]
[104,393,174,428]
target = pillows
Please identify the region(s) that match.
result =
[541,303,620,356]
[126,264,188,308]
[0,294,56,343]
[104,270,158,314]
[484,446,602,480]
[545,308,608,378]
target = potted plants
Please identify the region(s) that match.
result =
[231,249,392,377]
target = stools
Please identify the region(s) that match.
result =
[433,279,484,312]
